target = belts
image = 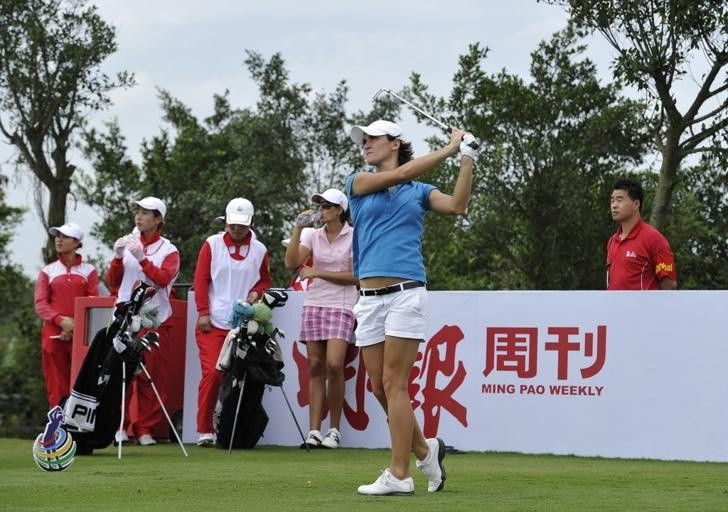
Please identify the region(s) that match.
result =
[359,280,425,296]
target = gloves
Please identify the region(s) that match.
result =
[459,133,479,161]
[124,233,147,264]
[112,236,127,260]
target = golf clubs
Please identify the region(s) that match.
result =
[111,281,188,457]
[225,328,312,453]
[372,87,479,149]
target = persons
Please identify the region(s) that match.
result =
[104,197,180,444]
[214,214,228,233]
[32,222,98,432]
[604,182,676,289]
[343,118,480,495]
[281,227,315,291]
[284,187,360,449]
[192,197,272,446]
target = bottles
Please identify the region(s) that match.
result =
[298,211,323,225]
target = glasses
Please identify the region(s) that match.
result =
[316,203,336,210]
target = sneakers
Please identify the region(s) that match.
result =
[357,468,415,497]
[135,434,158,446]
[115,430,129,443]
[197,432,218,446]
[299,430,322,449]
[416,437,447,493]
[320,428,342,449]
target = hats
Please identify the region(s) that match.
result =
[49,223,85,241]
[349,120,410,146]
[129,196,167,220]
[311,188,349,213]
[225,197,255,227]
